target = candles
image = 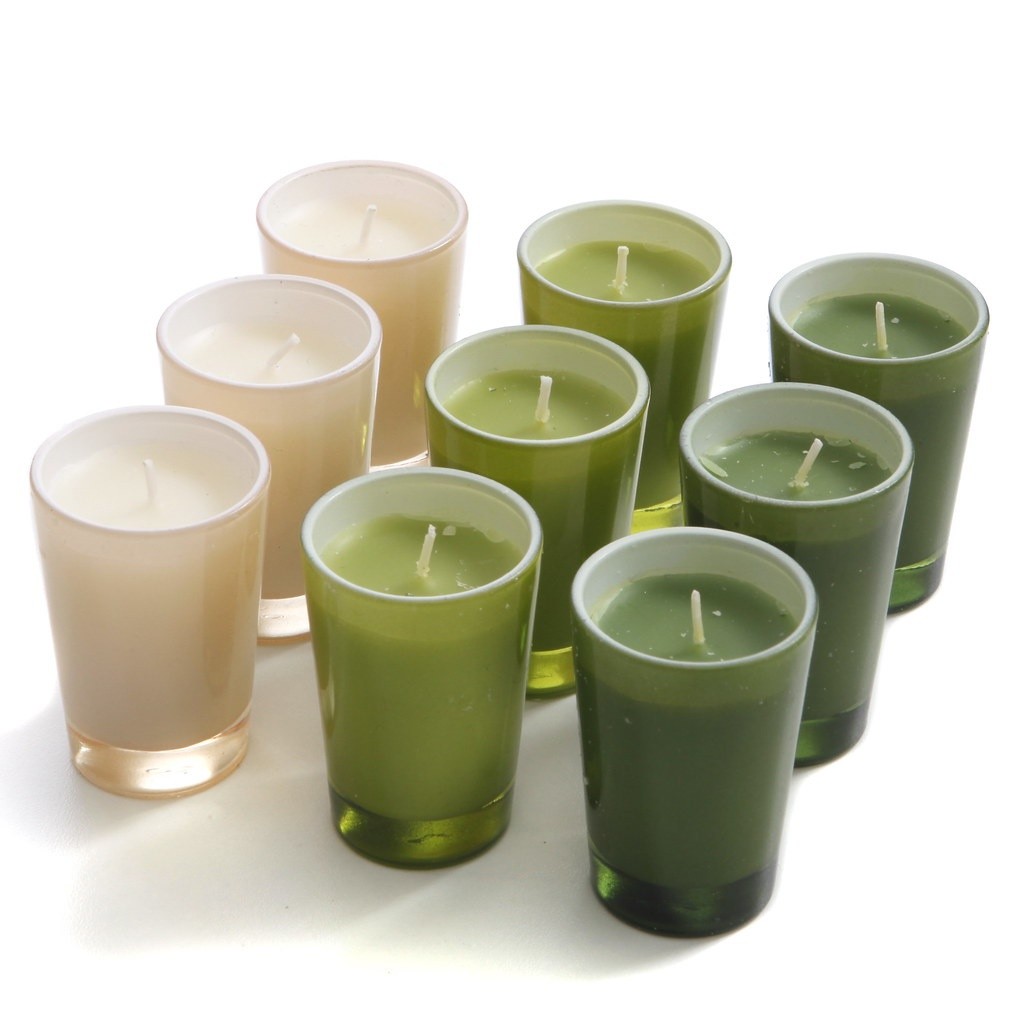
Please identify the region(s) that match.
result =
[31,161,987,940]
